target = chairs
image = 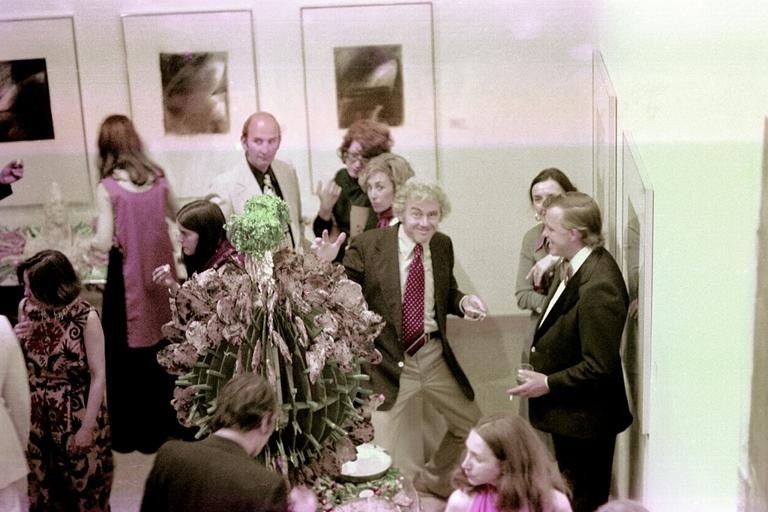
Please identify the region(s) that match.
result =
[421,308,532,467]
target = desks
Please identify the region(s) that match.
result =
[0,202,110,286]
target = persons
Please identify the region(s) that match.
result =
[0,314,31,512]
[508,192,633,512]
[140,372,288,512]
[0,158,24,201]
[597,501,649,512]
[444,410,573,512]
[89,115,183,455]
[358,153,414,265]
[514,167,579,417]
[152,201,244,298]
[312,120,393,263]
[623,200,639,500]
[311,177,486,502]
[13,250,114,512]
[204,112,303,254]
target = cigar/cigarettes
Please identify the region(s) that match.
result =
[510,394,513,401]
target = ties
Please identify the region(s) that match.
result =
[262,175,276,196]
[402,246,425,357]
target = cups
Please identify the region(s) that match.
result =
[512,362,534,387]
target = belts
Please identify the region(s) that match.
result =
[425,331,440,340]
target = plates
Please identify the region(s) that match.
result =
[337,444,392,481]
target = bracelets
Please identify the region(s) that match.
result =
[167,284,180,298]
